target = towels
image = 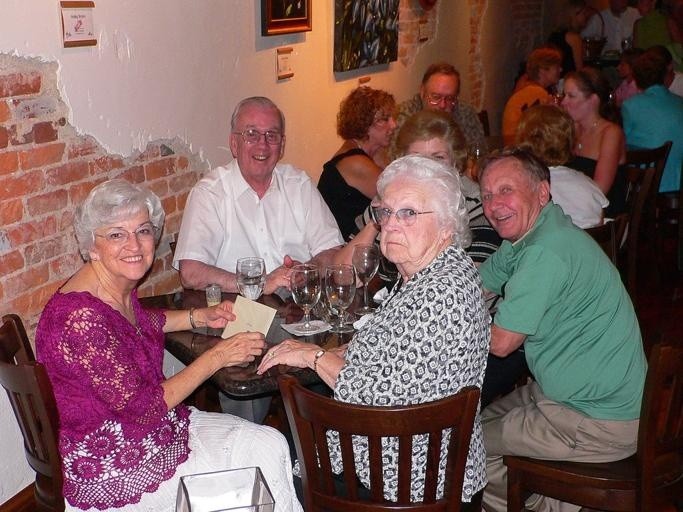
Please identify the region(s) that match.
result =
[190,314,294,345]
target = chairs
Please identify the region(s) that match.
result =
[502,343,681,511]
[660,173,680,270]
[624,140,672,241]
[0,314,65,512]
[610,167,652,310]
[479,110,490,137]
[276,372,480,512]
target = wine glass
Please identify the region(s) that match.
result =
[585,36,592,58]
[472,145,485,162]
[290,265,321,333]
[622,38,631,52]
[236,257,266,302]
[609,89,616,105]
[325,264,356,333]
[352,242,380,317]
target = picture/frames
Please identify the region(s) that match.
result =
[261,3,311,36]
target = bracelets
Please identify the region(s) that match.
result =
[188,305,199,332]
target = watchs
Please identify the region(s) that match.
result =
[312,347,330,371]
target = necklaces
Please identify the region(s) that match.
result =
[94,259,144,338]
[575,115,605,153]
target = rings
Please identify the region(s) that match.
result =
[265,349,275,359]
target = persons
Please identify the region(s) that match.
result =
[171,95,346,296]
[349,105,502,282]
[621,49,682,192]
[559,66,631,220]
[255,153,492,512]
[478,144,649,512]
[579,0,643,55]
[316,86,400,242]
[497,103,615,230]
[500,47,564,151]
[633,2,683,74]
[546,3,604,77]
[511,59,559,97]
[34,177,305,512]
[611,49,641,109]
[381,61,488,181]
[646,43,682,97]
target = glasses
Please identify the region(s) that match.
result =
[427,95,458,106]
[234,130,285,145]
[372,208,435,227]
[96,221,160,243]
